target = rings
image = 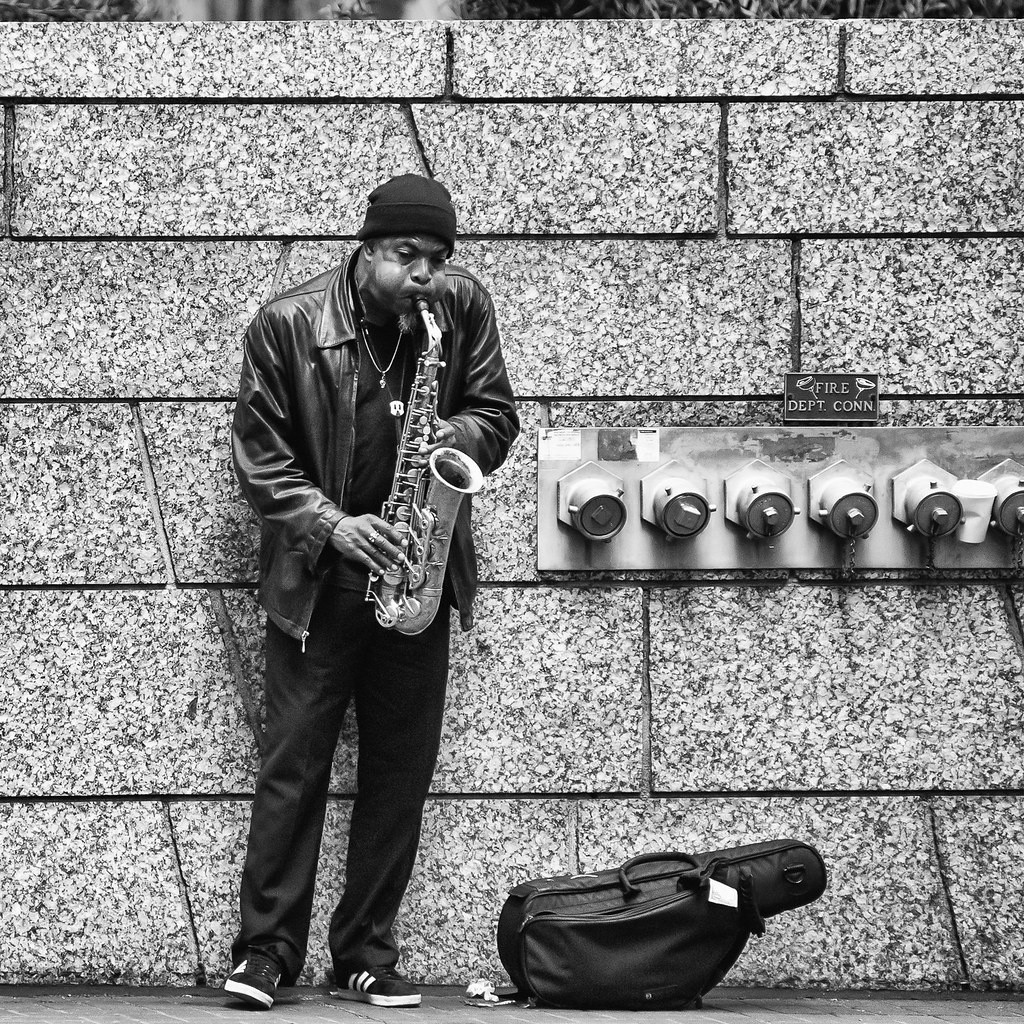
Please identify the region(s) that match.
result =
[366,528,381,545]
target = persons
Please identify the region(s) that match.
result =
[222,173,519,1009]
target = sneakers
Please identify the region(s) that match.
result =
[225,954,282,1007]
[336,964,422,1007]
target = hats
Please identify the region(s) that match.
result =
[356,173,457,257]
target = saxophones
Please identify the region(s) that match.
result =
[364,293,482,640]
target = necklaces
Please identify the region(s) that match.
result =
[361,321,403,391]
